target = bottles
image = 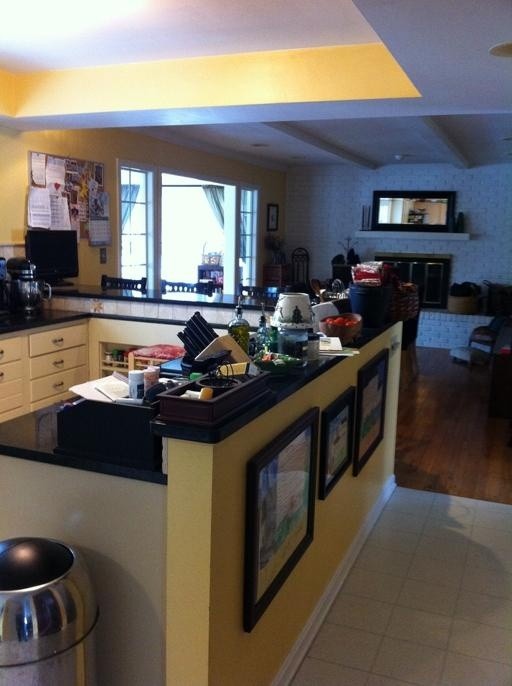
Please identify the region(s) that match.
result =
[256,302,269,350]
[227,294,250,356]
[127,365,161,399]
[104,350,124,363]
[456,212,464,232]
[276,328,309,368]
[269,326,278,352]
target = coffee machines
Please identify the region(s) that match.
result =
[5,257,52,318]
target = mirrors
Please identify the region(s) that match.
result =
[369,189,455,234]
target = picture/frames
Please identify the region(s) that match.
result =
[266,203,279,231]
[244,406,320,632]
[321,385,354,499]
[355,347,389,476]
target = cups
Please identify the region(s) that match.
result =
[362,205,371,230]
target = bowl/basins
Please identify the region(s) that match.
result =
[318,312,363,346]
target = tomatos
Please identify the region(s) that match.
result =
[326,316,358,326]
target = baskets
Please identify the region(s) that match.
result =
[392,285,419,318]
[447,293,476,314]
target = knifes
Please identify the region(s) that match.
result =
[176,311,219,357]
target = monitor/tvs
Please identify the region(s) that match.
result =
[25,230,79,287]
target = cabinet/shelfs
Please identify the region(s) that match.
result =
[376,256,450,310]
[101,353,170,379]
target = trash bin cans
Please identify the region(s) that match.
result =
[0,536,101,686]
